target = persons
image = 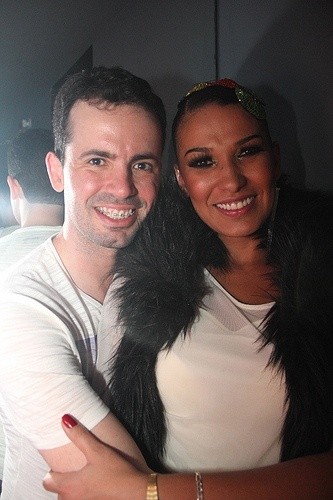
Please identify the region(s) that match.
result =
[41,79,333,500]
[0,64,168,500]
[0,128,65,275]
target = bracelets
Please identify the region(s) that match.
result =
[193,471,204,500]
[146,472,159,500]
